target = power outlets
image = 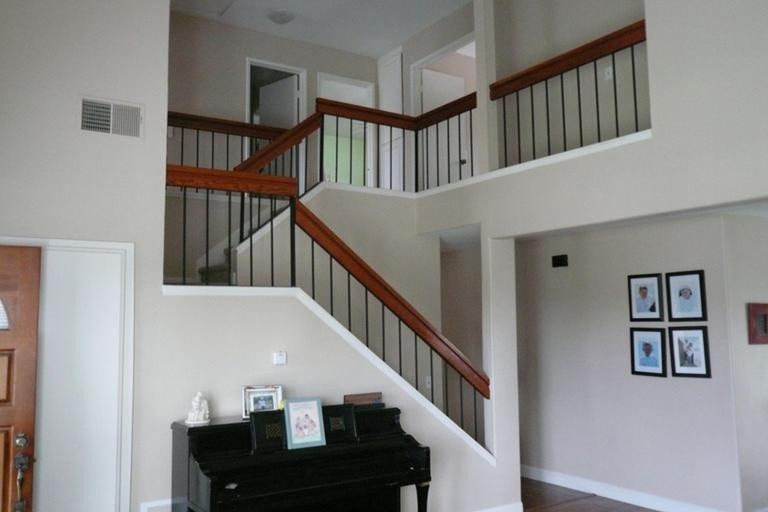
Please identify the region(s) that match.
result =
[272,351,288,365]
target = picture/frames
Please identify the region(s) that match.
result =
[746,301,768,345]
[668,325,712,378]
[626,272,665,322]
[665,269,708,323]
[629,326,667,377]
[241,384,282,419]
[283,396,327,450]
